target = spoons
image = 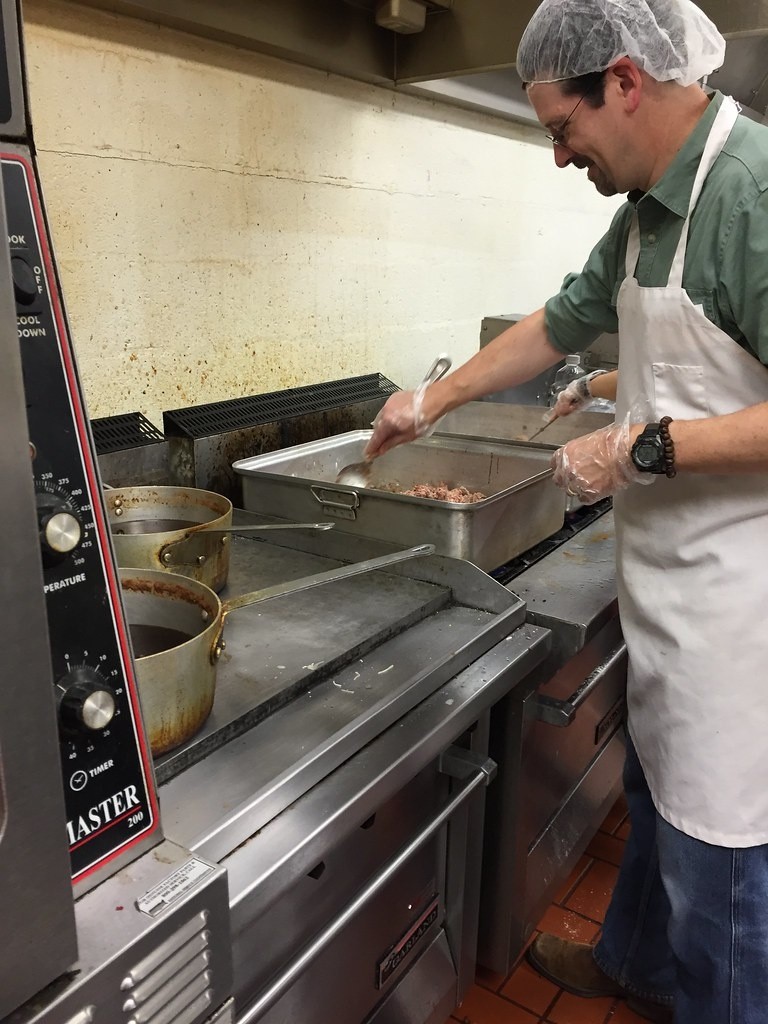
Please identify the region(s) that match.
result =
[335,357,453,491]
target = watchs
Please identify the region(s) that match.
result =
[631,423,665,474]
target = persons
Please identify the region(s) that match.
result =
[365,0,768,1023]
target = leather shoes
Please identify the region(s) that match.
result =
[526,932,676,1024]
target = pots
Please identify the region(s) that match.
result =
[100,486,336,595]
[117,543,438,756]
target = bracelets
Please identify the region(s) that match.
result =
[581,370,606,396]
[660,416,673,478]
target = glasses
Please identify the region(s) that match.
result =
[545,71,604,151]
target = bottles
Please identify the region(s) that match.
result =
[555,355,586,408]
